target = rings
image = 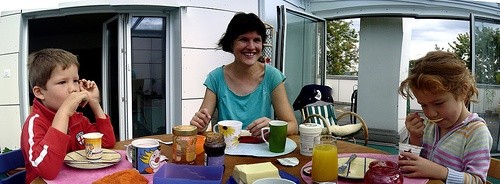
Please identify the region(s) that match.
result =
[196,118,199,121]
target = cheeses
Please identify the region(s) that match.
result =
[231,161,280,184]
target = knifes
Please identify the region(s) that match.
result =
[64,160,118,164]
[338,153,357,173]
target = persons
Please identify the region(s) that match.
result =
[21,50,116,184]
[397,51,493,184]
[189,12,298,139]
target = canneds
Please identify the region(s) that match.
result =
[364,160,404,184]
[172,125,198,166]
[204,132,226,174]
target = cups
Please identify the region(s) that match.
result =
[126,138,160,174]
[299,123,322,156]
[312,135,338,184]
[83,132,103,159]
[213,120,242,149]
[399,143,424,174]
[261,119,288,152]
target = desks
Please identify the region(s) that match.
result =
[30,130,445,184]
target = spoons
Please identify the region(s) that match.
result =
[157,139,173,144]
[411,114,443,122]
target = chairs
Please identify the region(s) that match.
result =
[0,149,26,184]
[299,84,368,147]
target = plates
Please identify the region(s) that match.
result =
[337,157,378,178]
[64,149,121,169]
